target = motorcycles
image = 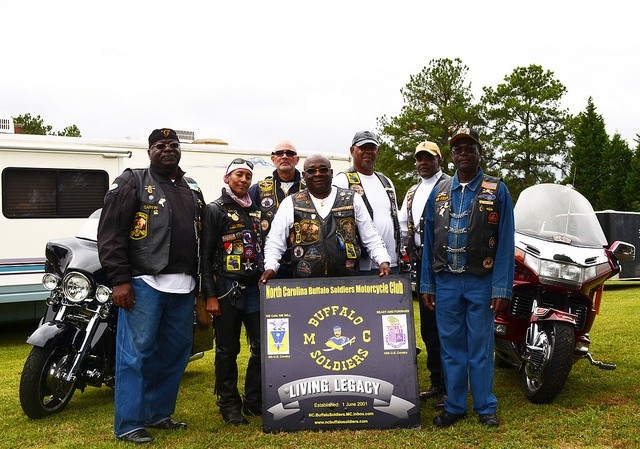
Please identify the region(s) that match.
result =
[20,206,203,418]
[494,182,636,403]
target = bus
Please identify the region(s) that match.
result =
[0,132,349,322]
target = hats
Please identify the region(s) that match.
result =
[415,141,441,157]
[149,128,179,149]
[223,158,253,183]
[450,128,479,144]
[352,131,378,146]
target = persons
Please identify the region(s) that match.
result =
[248,143,307,236]
[399,142,457,408]
[332,129,404,275]
[258,157,391,289]
[201,158,274,425]
[325,324,350,352]
[419,134,514,428]
[98,128,210,441]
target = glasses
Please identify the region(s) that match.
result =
[275,150,297,156]
[226,158,253,173]
[305,167,328,173]
[150,143,180,148]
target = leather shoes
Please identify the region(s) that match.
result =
[220,406,248,424]
[420,386,445,399]
[434,394,447,410]
[433,410,467,426]
[153,418,187,430]
[479,414,500,425]
[243,402,262,416]
[120,429,152,443]
